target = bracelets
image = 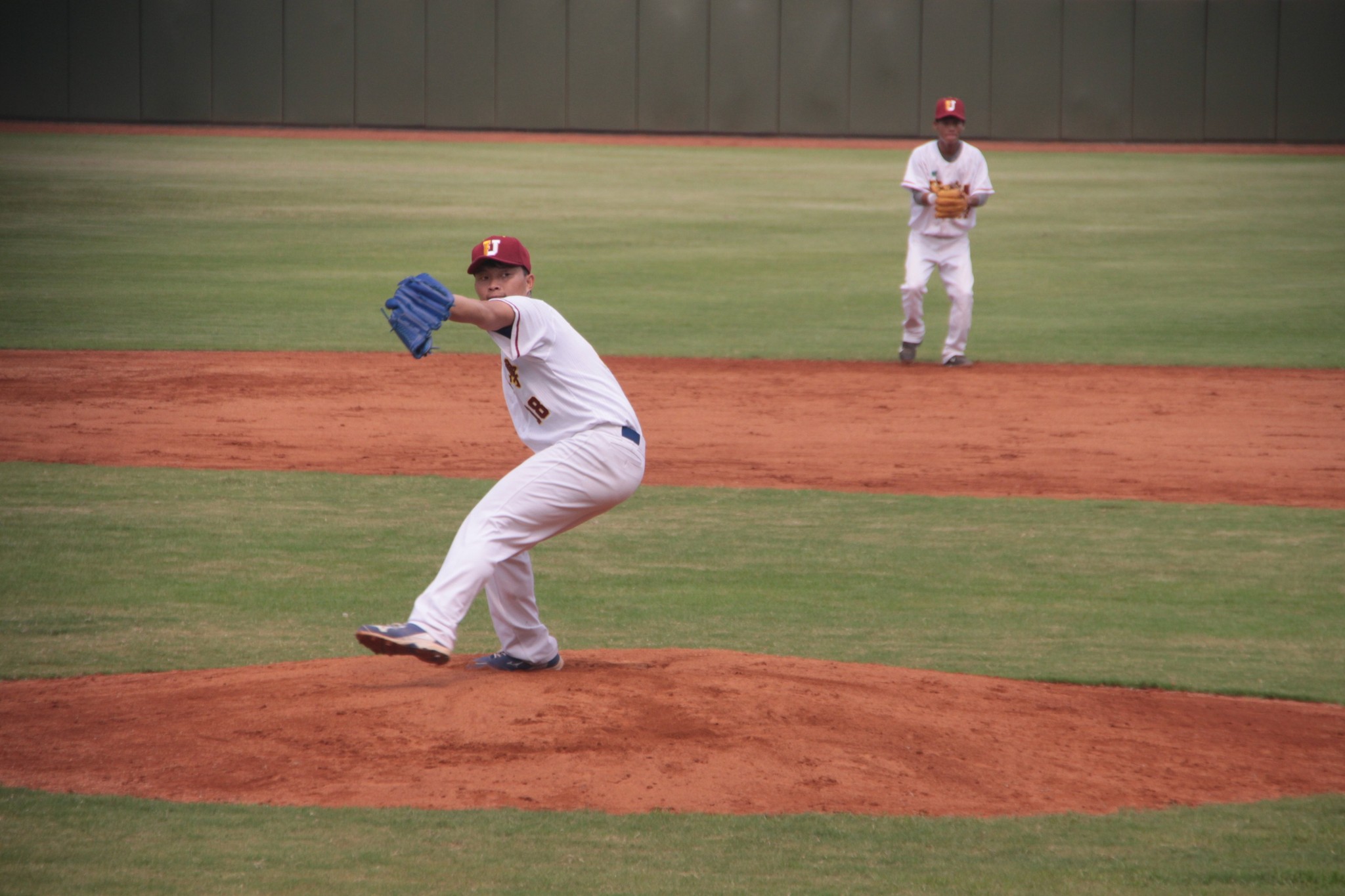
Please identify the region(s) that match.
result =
[928,193,937,204]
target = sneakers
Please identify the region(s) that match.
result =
[355,618,452,665]
[896,340,917,366]
[466,647,565,675]
[943,355,975,368]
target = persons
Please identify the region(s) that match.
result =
[899,97,995,366]
[357,237,646,671]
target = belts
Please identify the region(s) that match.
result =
[620,425,640,446]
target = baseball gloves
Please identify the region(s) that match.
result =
[931,184,969,219]
[378,270,457,359]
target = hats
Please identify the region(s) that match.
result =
[931,99,967,124]
[466,235,533,273]
